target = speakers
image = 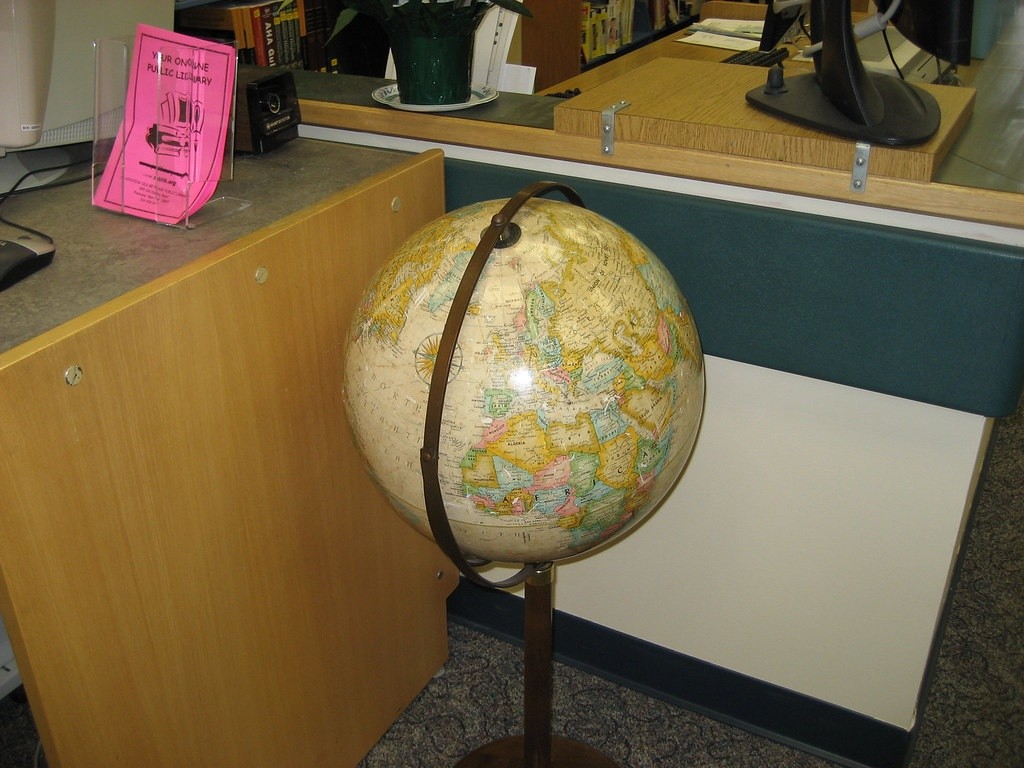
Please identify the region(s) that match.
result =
[236,63,300,155]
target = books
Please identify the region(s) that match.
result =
[188,0,303,70]
[580,0,679,64]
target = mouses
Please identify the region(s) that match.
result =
[0,234,57,292]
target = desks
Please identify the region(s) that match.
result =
[0,137,462,768]
[533,1,816,99]
[553,58,977,184]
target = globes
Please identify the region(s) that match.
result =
[341,179,704,768]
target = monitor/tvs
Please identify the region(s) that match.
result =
[0,0,176,195]
[747,0,976,146]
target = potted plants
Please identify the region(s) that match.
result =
[321,0,532,105]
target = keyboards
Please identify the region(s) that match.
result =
[719,46,789,68]
